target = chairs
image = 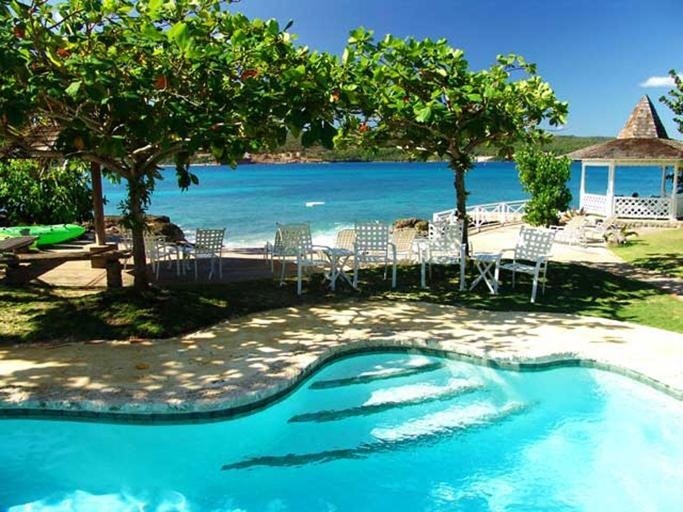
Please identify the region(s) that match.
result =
[492,224,558,303]
[117,223,226,281]
[264,217,467,295]
[549,211,620,248]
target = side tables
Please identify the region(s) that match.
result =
[469,255,501,295]
